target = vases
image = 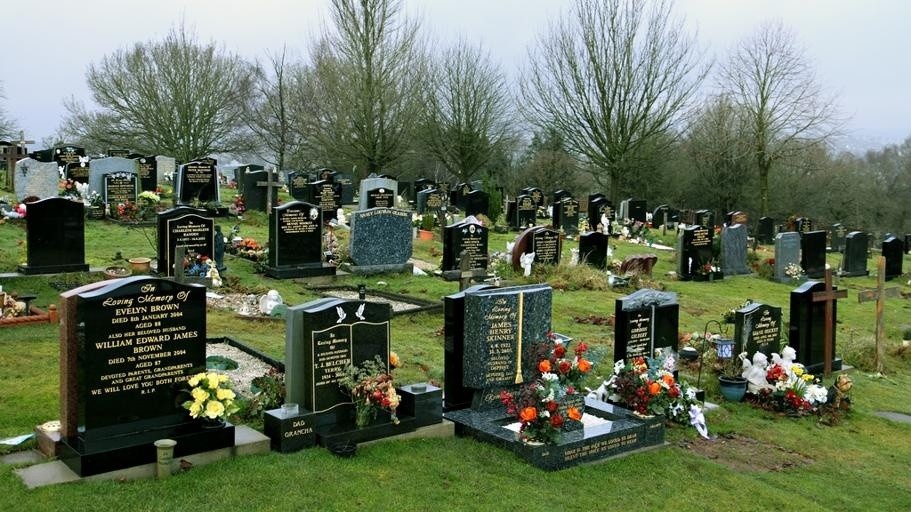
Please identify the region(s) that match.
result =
[87,205,104,220]
[103,266,132,279]
[128,258,151,274]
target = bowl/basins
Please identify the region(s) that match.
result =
[330,442,358,458]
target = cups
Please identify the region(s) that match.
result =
[153,438,178,479]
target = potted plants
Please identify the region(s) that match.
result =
[902,327,910,347]
[412,219,420,239]
[419,214,435,240]
[718,358,749,403]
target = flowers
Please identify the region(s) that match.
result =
[337,356,402,425]
[502,341,591,445]
[87,191,104,207]
[178,369,243,421]
